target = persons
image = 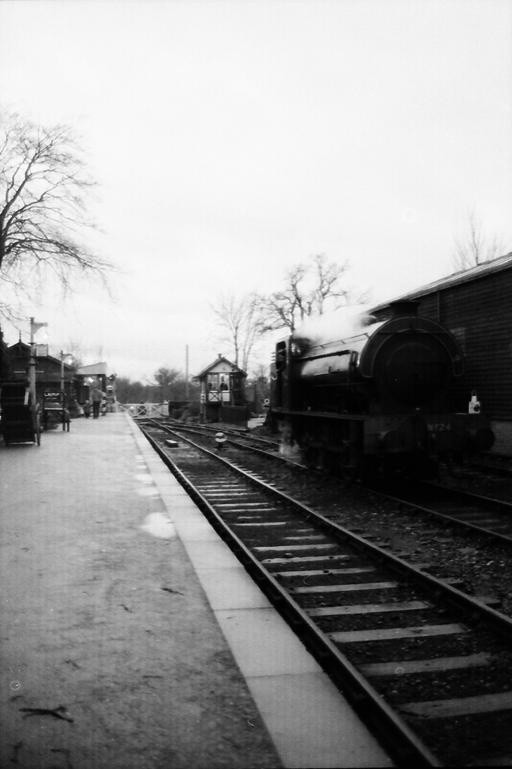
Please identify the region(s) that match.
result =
[219,379,229,391]
[92,386,103,420]
[83,400,93,418]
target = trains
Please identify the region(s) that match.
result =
[270,244,511,522]
